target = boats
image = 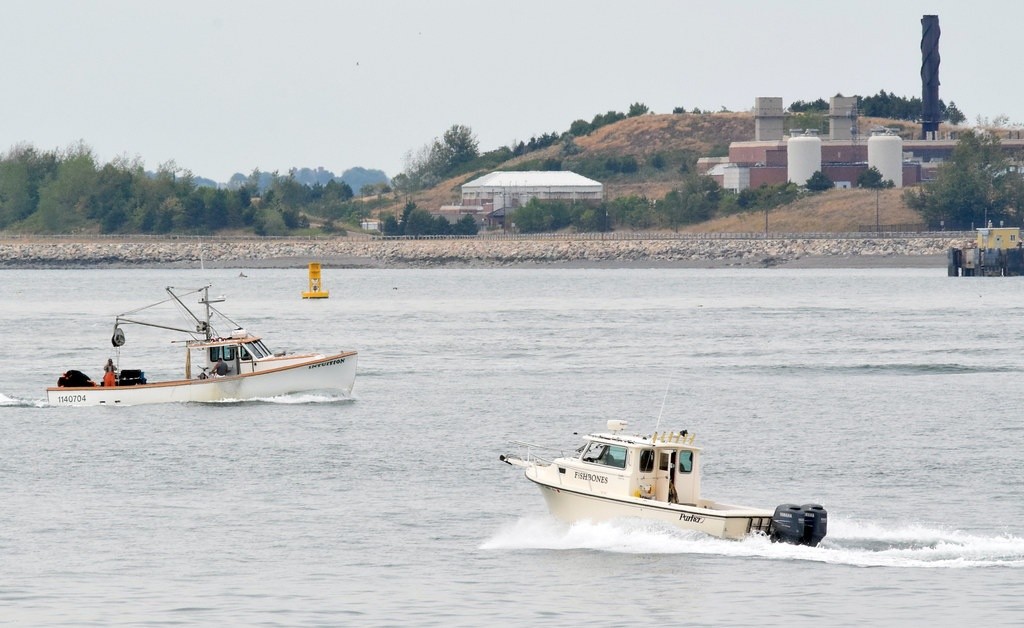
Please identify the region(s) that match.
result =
[44,250,360,408]
[499,380,829,548]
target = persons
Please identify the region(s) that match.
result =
[104,359,117,386]
[212,358,229,377]
